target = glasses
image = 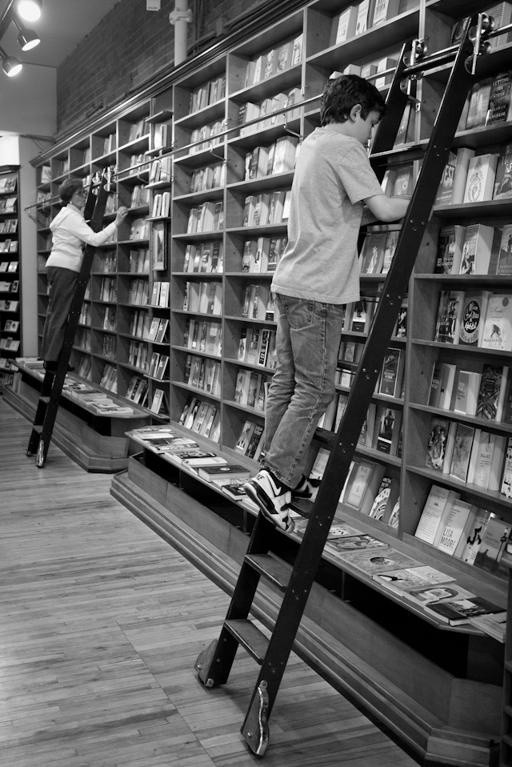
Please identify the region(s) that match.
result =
[74,188,88,195]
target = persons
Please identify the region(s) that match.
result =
[42,178,128,377]
[242,75,411,533]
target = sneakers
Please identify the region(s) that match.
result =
[43,360,77,372]
[261,461,314,498]
[248,469,295,535]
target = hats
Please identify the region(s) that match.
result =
[58,176,82,201]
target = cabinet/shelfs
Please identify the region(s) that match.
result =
[109,2,511,767]
[1,103,169,473]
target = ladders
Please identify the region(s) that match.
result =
[191,12,495,757]
[26,166,113,468]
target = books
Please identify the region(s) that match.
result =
[20,73,223,443]
[414,0,512,583]
[287,514,508,641]
[2,176,22,387]
[135,427,305,523]
[226,2,416,526]
